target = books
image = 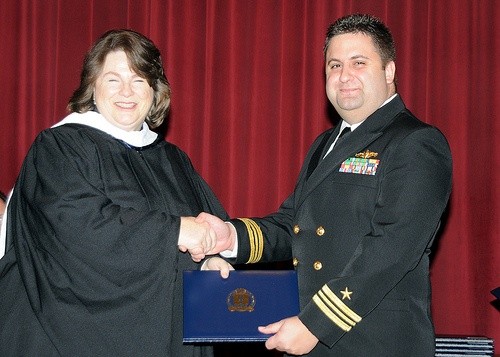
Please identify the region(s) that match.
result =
[432,333,494,356]
[182,269,301,344]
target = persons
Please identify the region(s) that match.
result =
[179,14,453,357]
[0,29,235,357]
